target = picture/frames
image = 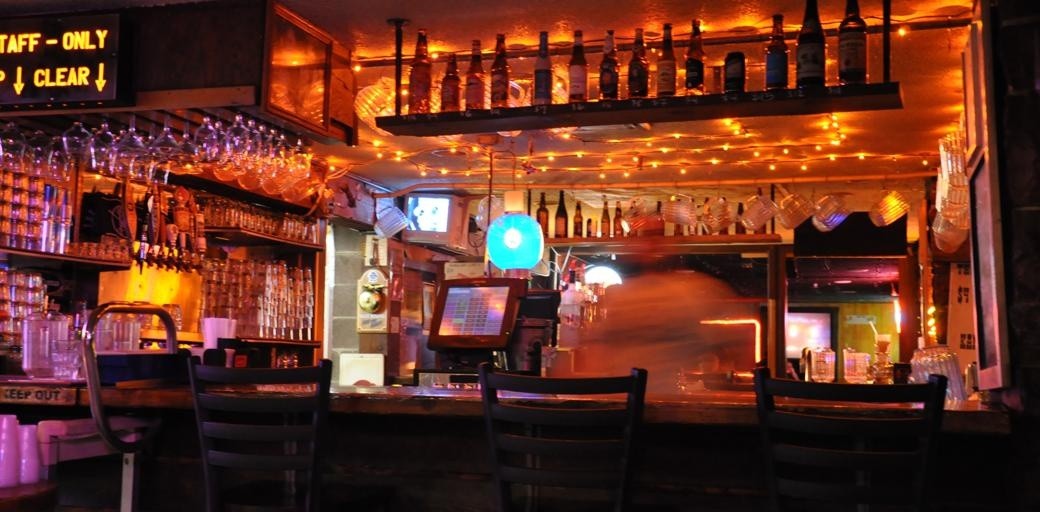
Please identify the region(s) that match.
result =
[259,3,337,137]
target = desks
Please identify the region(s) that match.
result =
[2,377,1040,512]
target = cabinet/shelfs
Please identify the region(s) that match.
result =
[0,0,358,512]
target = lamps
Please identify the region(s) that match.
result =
[485,130,547,282]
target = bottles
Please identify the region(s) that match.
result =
[711,65,721,92]
[628,202,637,237]
[532,32,552,105]
[568,31,587,103]
[735,202,747,235]
[599,31,618,100]
[466,40,487,110]
[490,33,510,110]
[628,28,648,99]
[554,191,567,238]
[409,29,431,115]
[654,202,665,236]
[754,188,766,234]
[561,270,583,346]
[657,24,676,97]
[766,15,788,90]
[674,200,684,235]
[601,200,610,237]
[686,20,703,96]
[718,198,728,234]
[796,0,825,88]
[688,198,698,236]
[724,52,745,92]
[573,200,582,238]
[614,202,623,236]
[837,1,871,84]
[703,197,713,236]
[536,192,548,238]
[441,54,460,112]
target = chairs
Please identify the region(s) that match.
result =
[477,361,648,512]
[187,353,334,512]
[754,366,948,512]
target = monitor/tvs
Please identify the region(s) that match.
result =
[427,277,529,351]
[759,304,839,379]
[513,289,561,349]
[400,192,470,248]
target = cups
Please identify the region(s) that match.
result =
[112,321,132,353]
[218,319,237,340]
[0,119,27,166]
[811,350,835,382]
[868,189,911,227]
[160,303,183,330]
[90,121,115,152]
[930,129,969,257]
[816,194,850,229]
[132,321,141,354]
[94,318,113,353]
[740,195,779,230]
[116,112,144,151]
[18,425,39,485]
[372,205,410,241]
[245,119,262,154]
[623,198,658,230]
[191,115,218,146]
[877,334,892,352]
[660,192,698,225]
[130,153,152,180]
[48,151,75,181]
[203,317,218,349]
[201,258,314,340]
[844,351,870,383]
[585,283,606,320]
[27,122,55,169]
[60,119,91,157]
[776,194,815,230]
[0,415,19,488]
[149,114,180,150]
[226,112,250,152]
[699,196,740,236]
[51,339,82,380]
[475,195,504,231]
[209,208,318,244]
[22,319,70,380]
[680,353,704,389]
[909,347,965,402]
